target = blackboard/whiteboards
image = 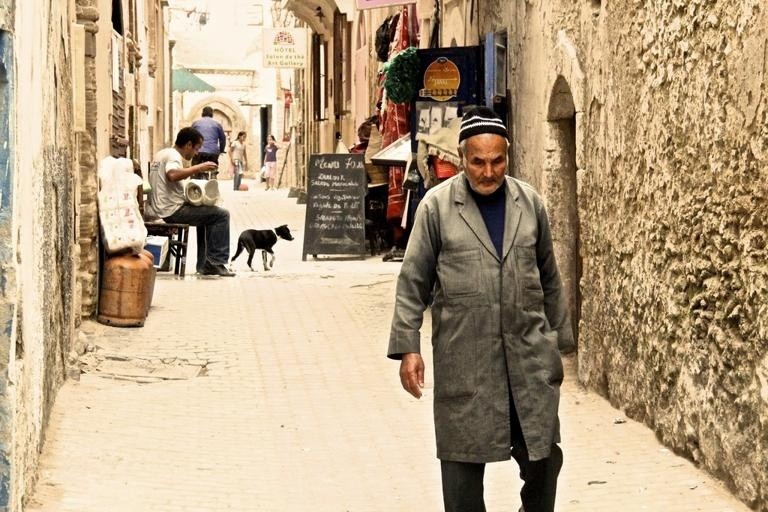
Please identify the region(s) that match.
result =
[303,153,366,254]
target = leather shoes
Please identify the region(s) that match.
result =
[196,260,236,276]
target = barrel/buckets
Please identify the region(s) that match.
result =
[97,249,157,326]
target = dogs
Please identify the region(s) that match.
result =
[231,223,295,271]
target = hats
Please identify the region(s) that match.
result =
[459,107,507,144]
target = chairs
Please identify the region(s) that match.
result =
[137,182,190,279]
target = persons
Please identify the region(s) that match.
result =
[382,103,580,512]
[146,126,237,278]
[228,131,248,193]
[262,133,282,191]
[188,105,227,181]
